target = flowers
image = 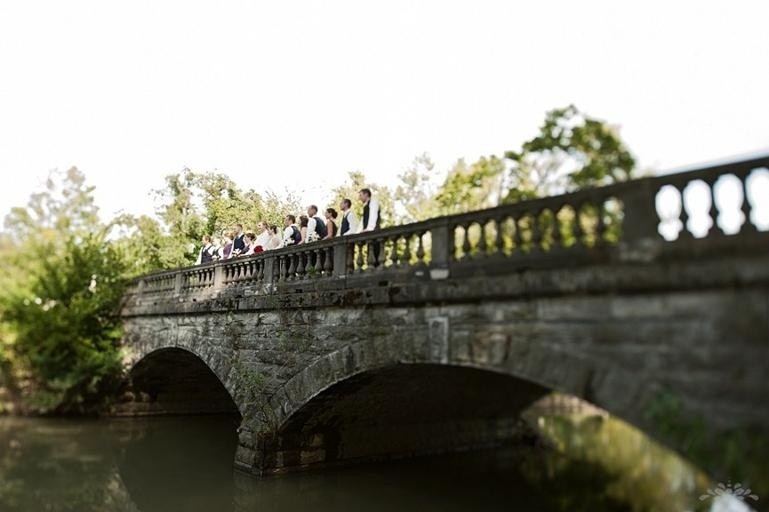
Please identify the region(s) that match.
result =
[232,248,241,258]
[253,246,264,253]
[305,231,320,243]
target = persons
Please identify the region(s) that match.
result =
[192,188,382,285]
